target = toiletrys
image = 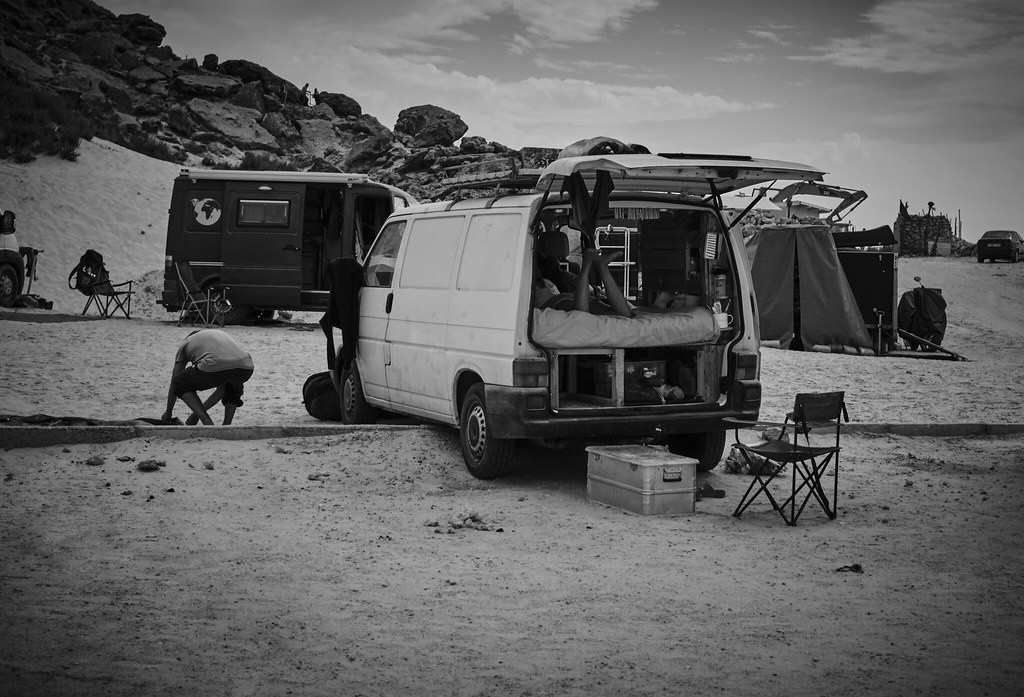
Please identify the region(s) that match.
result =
[711,299,722,313]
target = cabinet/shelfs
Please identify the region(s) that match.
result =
[549,347,625,408]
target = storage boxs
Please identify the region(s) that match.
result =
[593,360,667,400]
[585,444,700,515]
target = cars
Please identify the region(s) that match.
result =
[977,230,1024,263]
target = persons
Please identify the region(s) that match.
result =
[301,83,320,105]
[161,328,254,425]
[535,248,634,318]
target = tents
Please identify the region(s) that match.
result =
[750,223,875,352]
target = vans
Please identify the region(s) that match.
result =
[330,135,830,478]
[0,208,25,307]
[157,167,420,324]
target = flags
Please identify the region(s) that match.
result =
[900,201,912,221]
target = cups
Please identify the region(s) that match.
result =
[715,274,726,297]
[713,313,733,328]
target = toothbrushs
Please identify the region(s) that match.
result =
[706,304,713,314]
[724,299,732,312]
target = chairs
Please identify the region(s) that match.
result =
[731,390,850,526]
[175,261,225,327]
[82,270,136,319]
[538,231,581,292]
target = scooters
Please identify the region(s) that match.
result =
[900,276,947,352]
